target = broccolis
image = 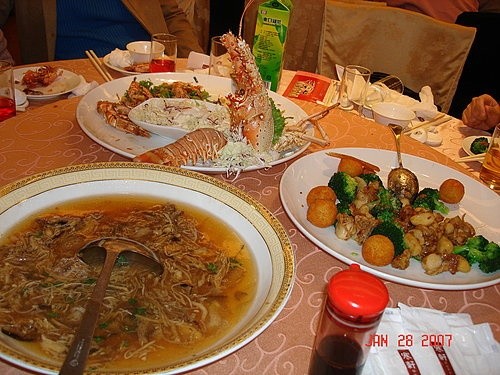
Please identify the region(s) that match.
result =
[329,172,500,275]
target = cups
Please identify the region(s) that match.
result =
[337,65,371,115]
[479,123,500,194]
[150,34,177,72]
[310,264,389,374]
[1,61,18,123]
[366,75,404,107]
[209,38,232,76]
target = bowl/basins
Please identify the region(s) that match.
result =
[369,102,415,130]
[0,161,295,374]
[126,41,165,63]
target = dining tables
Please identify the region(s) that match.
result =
[0,56,499,375]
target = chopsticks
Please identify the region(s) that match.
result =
[452,152,486,164]
[85,48,113,83]
[401,114,450,136]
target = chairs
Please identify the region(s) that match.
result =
[316,1,478,115]
[42,1,208,62]
[243,1,386,78]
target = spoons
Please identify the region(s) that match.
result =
[387,123,421,203]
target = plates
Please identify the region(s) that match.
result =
[461,134,491,163]
[101,54,145,75]
[76,71,314,170]
[11,66,81,100]
[280,147,500,292]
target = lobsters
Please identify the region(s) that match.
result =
[216,31,340,154]
[131,128,228,168]
[97,81,198,138]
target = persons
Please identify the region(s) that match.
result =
[439,0,499,134]
[0,0,204,76]
